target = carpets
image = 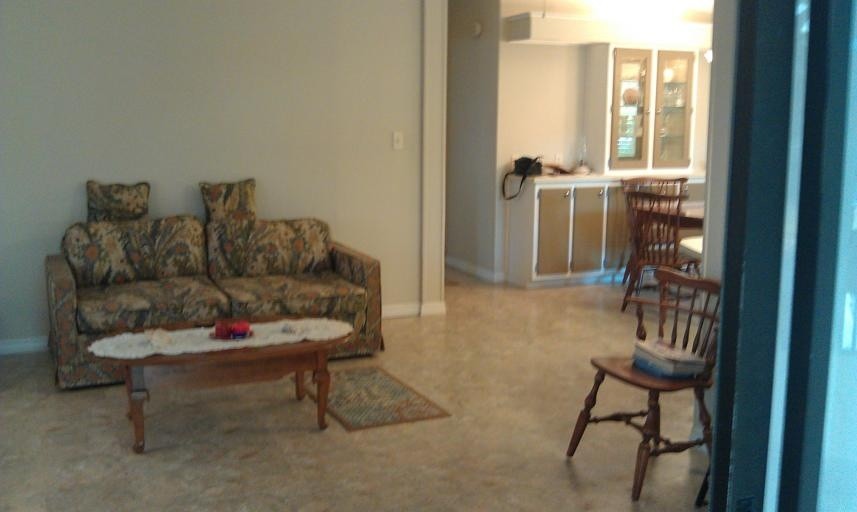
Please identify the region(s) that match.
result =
[290,367,449,431]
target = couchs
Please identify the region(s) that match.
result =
[44,241,384,390]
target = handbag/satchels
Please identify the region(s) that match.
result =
[515,157,542,175]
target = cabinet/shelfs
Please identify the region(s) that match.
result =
[507,42,707,290]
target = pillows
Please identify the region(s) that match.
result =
[62,178,335,289]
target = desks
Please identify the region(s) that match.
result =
[78,317,353,453]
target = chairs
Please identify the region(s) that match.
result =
[617,177,702,313]
[567,266,722,503]
